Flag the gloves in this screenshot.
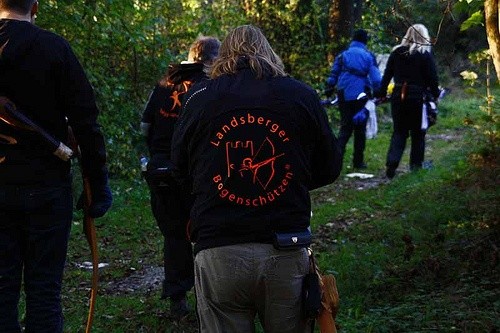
[379,89,388,98]
[324,86,335,98]
[373,92,380,99]
[75,171,113,219]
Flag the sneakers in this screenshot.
[353,163,368,169]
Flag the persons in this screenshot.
[323,28,383,169]
[170,26,343,333]
[379,24,441,178]
[140,36,223,320]
[0,0,112,333]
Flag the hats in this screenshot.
[353,29,369,44]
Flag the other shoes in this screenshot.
[409,163,424,171]
[386,161,396,180]
[172,307,193,323]
